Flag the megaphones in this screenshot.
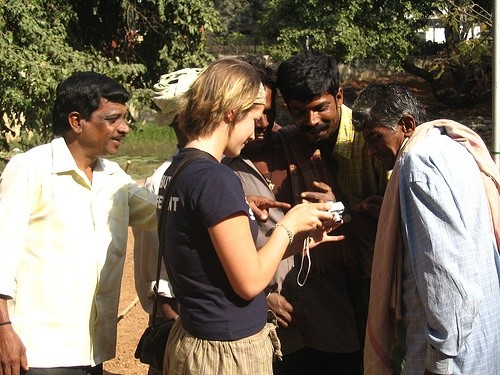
[319,201,345,224]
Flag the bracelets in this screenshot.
[0,71,157,374]
[271,223,295,245]
[1,320,12,327]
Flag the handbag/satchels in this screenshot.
[135,318,176,370]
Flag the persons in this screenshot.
[132,48,291,375]
[277,49,405,375]
[350,77,500,375]
